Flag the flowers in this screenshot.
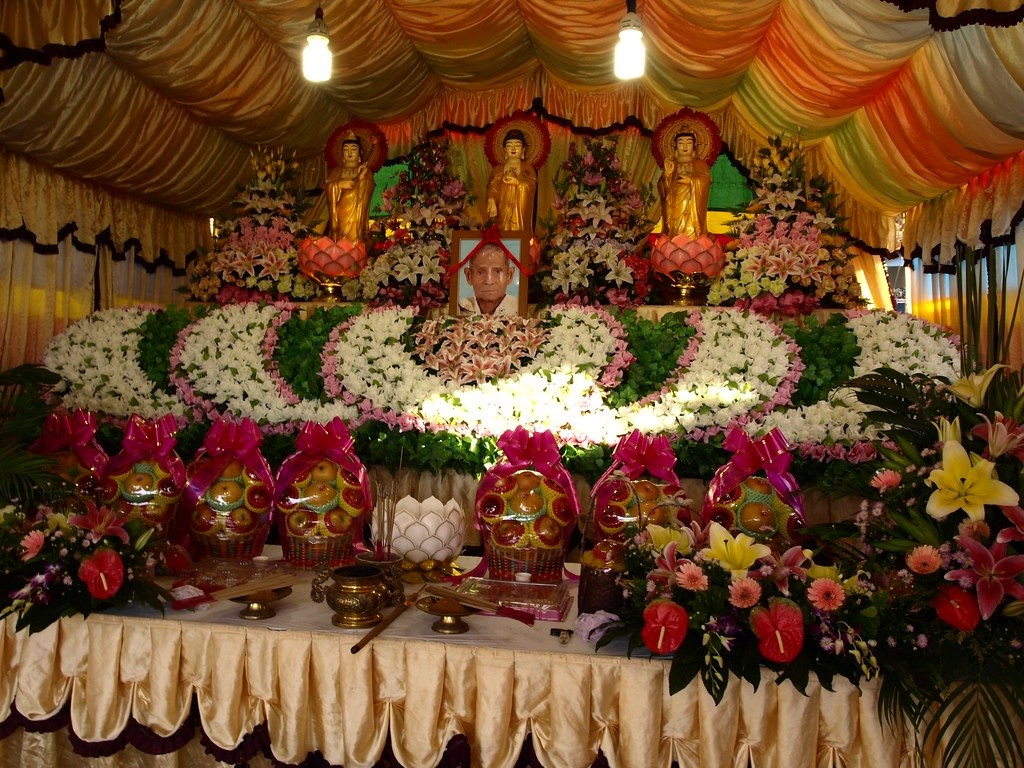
[588,518,880,707]
[0,498,195,638]
[705,127,871,318]
[341,136,468,306]
[827,363,1024,768]
[536,134,654,307]
[172,142,324,302]
[42,304,975,494]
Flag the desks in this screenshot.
[0,556,1024,768]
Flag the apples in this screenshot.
[46,445,368,536]
[477,472,805,565]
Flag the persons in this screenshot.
[486,129,537,231]
[323,130,370,243]
[457,242,518,317]
[657,124,710,241]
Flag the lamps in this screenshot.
[614,0,646,79]
[302,0,333,83]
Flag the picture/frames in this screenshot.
[448,229,530,319]
